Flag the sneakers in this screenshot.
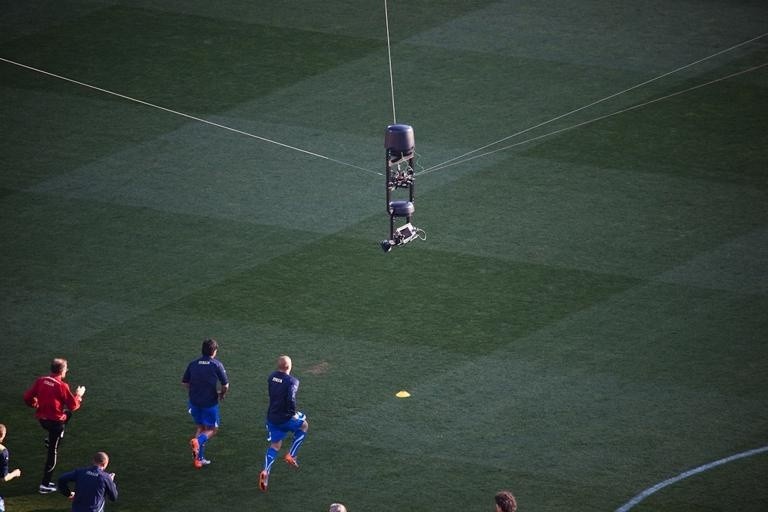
[44,437,49,449]
[191,438,200,460]
[39,481,57,495]
[195,459,211,468]
[285,453,298,468]
[259,470,268,492]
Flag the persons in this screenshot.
[494,491,517,510]
[257,354,309,491]
[22,358,87,495]
[0,421,23,511]
[181,339,230,470]
[54,452,119,512]
[329,503,347,512]
